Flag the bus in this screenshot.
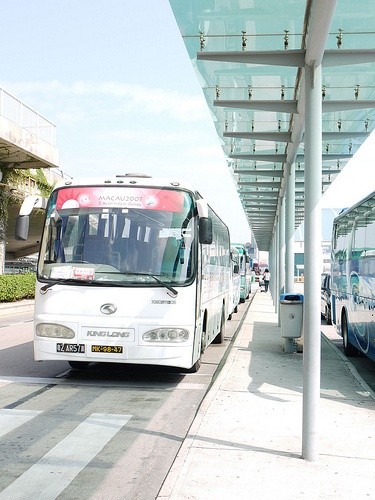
[232,240,254,316]
[34,173,231,375]
[328,191,374,354]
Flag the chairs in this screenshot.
[78,234,191,277]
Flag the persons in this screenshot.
[263,269,270,293]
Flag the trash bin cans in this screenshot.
[279,293,303,338]
